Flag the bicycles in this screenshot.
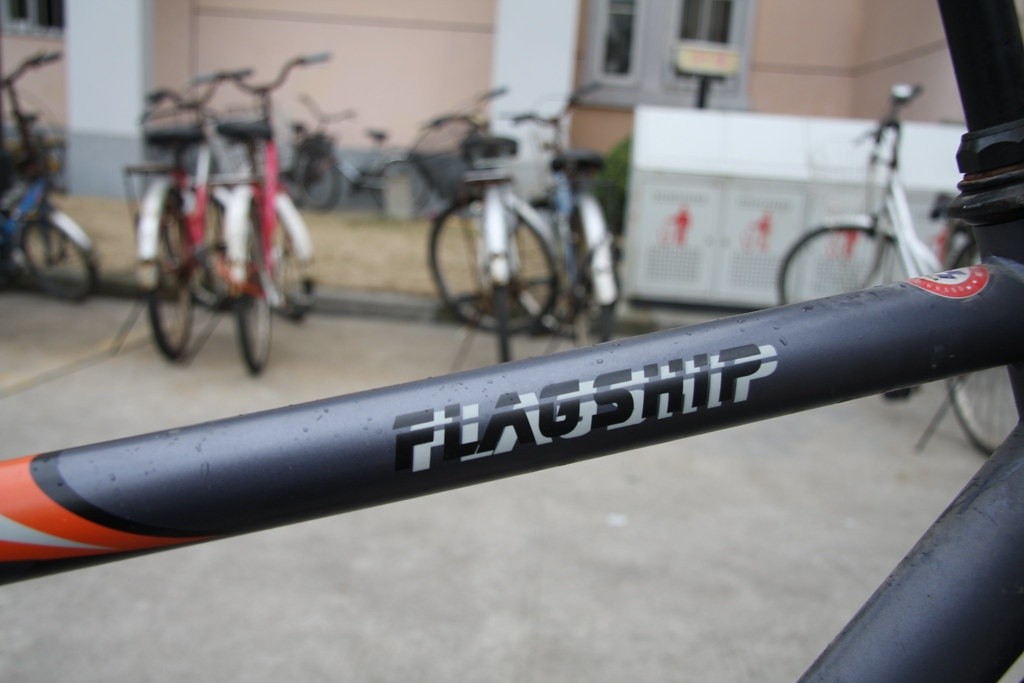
[282,90,433,222]
[375,84,562,363]
[112,41,343,378]
[506,79,619,343]
[775,81,1021,458]
[1,47,100,304]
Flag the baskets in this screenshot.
[142,104,219,175]
[410,119,481,204]
[202,106,294,174]
[306,133,333,155]
[11,138,63,184]
